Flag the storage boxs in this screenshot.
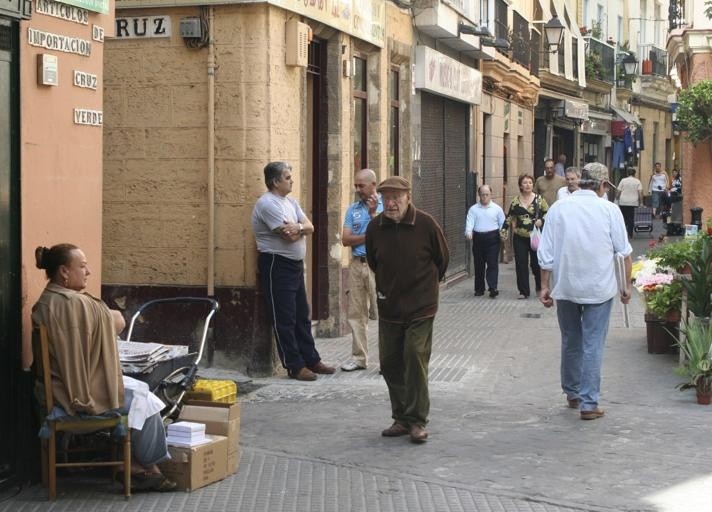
[158,435,228,491]
[176,400,240,476]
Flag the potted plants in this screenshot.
[644,283,681,355]
[658,317,712,405]
[647,236,699,275]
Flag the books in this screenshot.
[167,421,206,431]
[167,431,206,437]
[165,438,206,442]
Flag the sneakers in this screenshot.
[580,407,605,420]
[518,294,528,299]
[409,422,428,442]
[381,421,411,437]
[569,399,580,409]
[340,362,367,371]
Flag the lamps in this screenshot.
[505,14,565,59]
[609,52,640,83]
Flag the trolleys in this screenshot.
[632,206,655,240]
[121,293,221,430]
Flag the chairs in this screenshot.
[29,322,133,500]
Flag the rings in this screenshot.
[286,230,290,234]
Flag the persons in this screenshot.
[534,160,567,205]
[341,169,385,372]
[537,162,633,420]
[557,167,581,201]
[614,168,643,243]
[555,154,566,175]
[648,163,670,218]
[366,176,449,442]
[251,160,336,380]
[465,185,507,297]
[669,167,680,192]
[32,243,177,491]
[501,175,549,300]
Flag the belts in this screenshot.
[352,256,368,263]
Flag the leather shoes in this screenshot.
[288,368,318,381]
[114,471,167,491]
[310,361,336,374]
[474,291,484,296]
[155,478,179,490]
[489,289,499,298]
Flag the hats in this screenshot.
[581,161,617,189]
[375,176,412,192]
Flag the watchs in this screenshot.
[300,223,303,236]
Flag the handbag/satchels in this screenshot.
[500,247,513,264]
